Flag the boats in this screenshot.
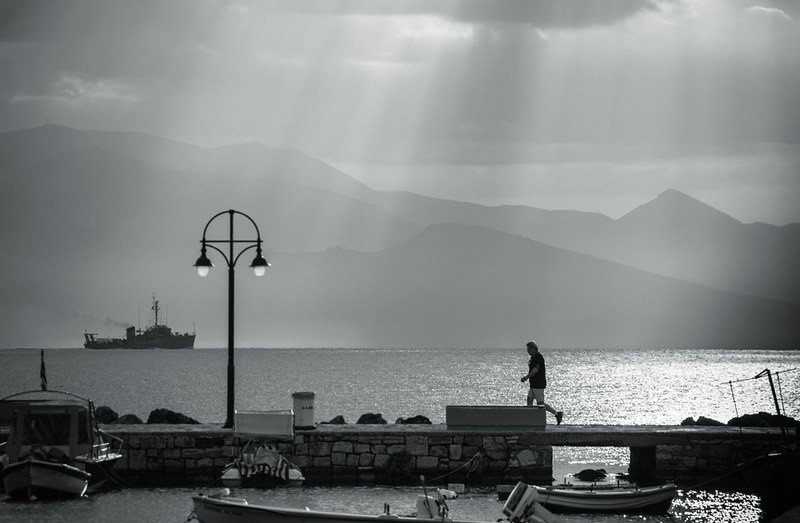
[495,465,679,517]
[183,474,491,523]
[0,346,124,501]
[83,291,196,350]
[1,447,92,502]
[218,435,306,489]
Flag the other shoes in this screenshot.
[554,412,563,425]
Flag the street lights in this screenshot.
[192,209,273,431]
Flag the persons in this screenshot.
[521,341,563,425]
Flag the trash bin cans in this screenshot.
[292,392,315,429]
